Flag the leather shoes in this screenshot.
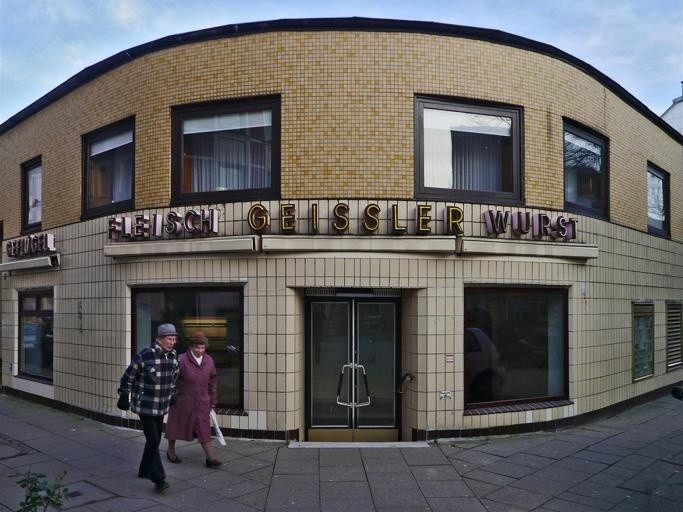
[155,480,169,492]
[205,458,222,468]
[167,451,182,463]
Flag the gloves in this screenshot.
[118,393,130,411]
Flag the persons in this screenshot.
[163,332,222,468]
[117,323,181,493]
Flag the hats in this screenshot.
[190,332,210,345]
[157,323,178,337]
